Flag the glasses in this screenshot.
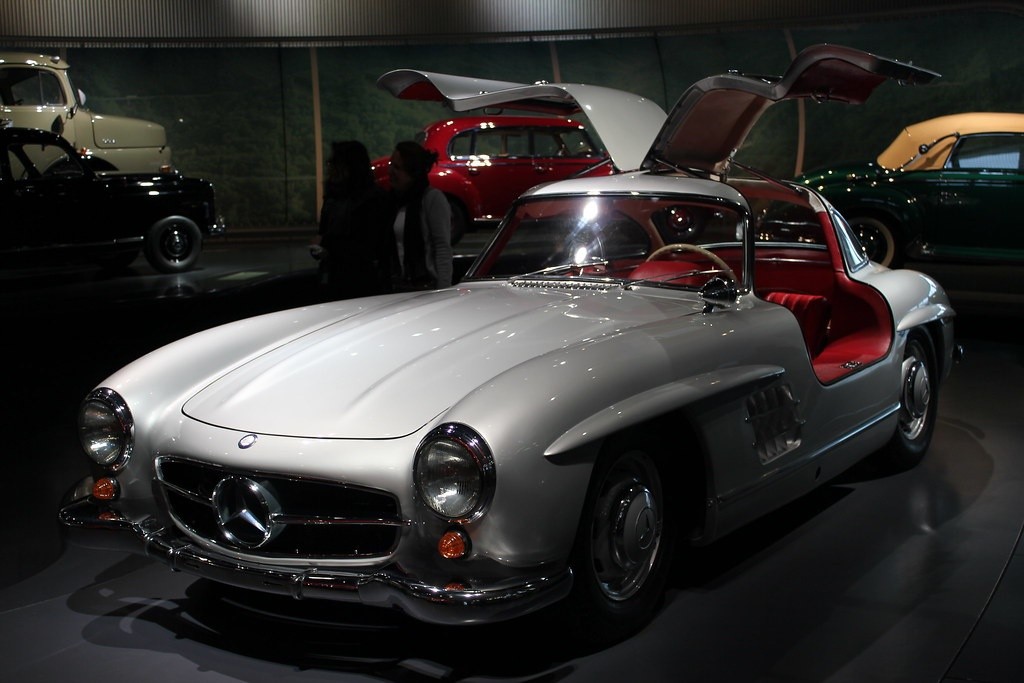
[325,159,345,168]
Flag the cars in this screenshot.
[366,113,617,244]
[1,128,226,279]
[758,110,1024,271]
[0,50,173,177]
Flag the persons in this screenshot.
[309,140,404,303]
[384,141,454,292]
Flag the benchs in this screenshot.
[688,256,858,343]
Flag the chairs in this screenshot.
[629,259,702,288]
[766,292,827,365]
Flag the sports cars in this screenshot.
[55,39,958,629]
